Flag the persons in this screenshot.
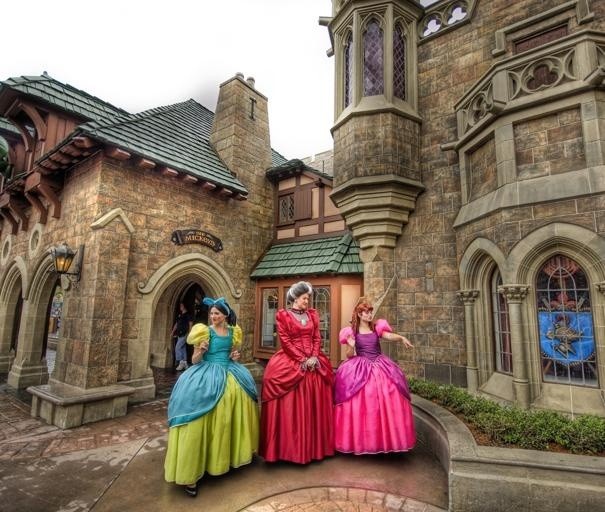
[163,297,260,498]
[192,295,208,326]
[332,297,419,457]
[259,281,337,466]
[168,301,196,372]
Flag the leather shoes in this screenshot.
[184,486,197,496]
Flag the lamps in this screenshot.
[44,241,84,281]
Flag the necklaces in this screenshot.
[291,308,308,327]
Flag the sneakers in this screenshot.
[175,360,189,371]
